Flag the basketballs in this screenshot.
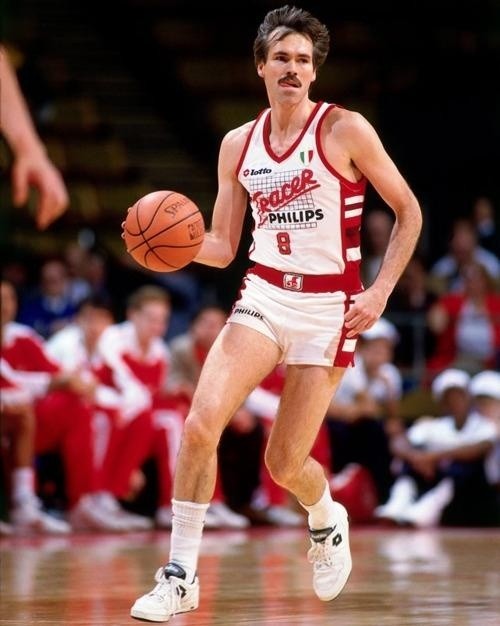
[124,190,205,273]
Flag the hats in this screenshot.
[359,317,499,398]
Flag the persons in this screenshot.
[0,192,498,537]
[127,8,425,622]
[0,45,72,230]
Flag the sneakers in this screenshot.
[0,501,71,537]
[129,563,200,621]
[307,501,353,602]
